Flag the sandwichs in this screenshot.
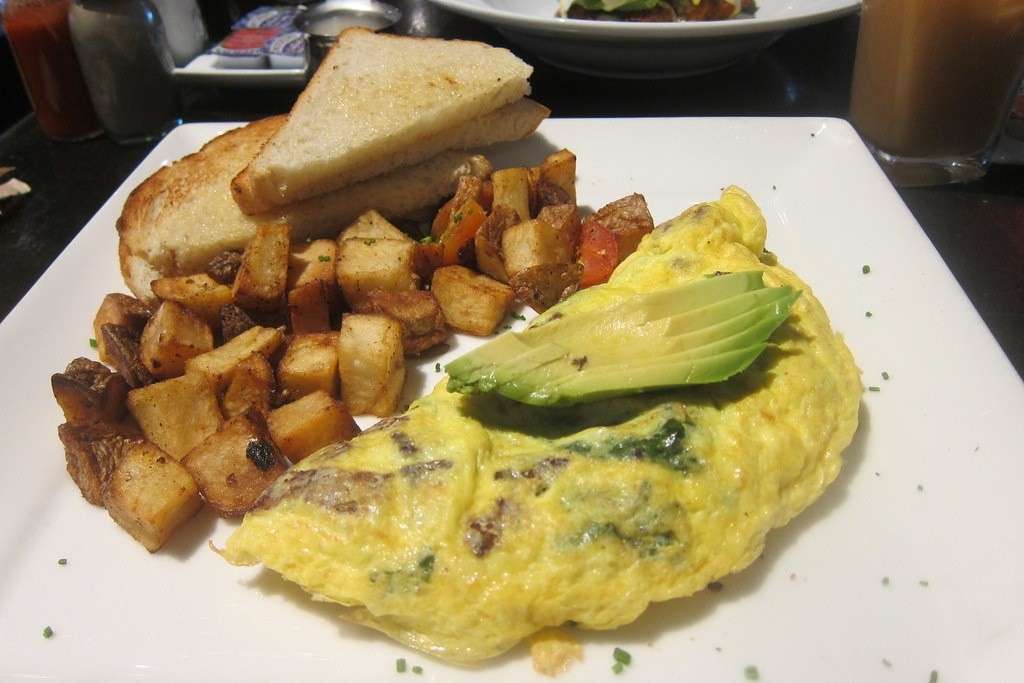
[113,22,551,310]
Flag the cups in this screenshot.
[294,1,403,86]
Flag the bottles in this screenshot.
[0,0,104,142]
[67,0,183,143]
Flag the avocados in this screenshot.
[443,269,802,404]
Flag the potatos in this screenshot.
[57,146,657,553]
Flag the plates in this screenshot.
[0,116,1024,683]
[433,0,863,78]
[171,46,304,90]
